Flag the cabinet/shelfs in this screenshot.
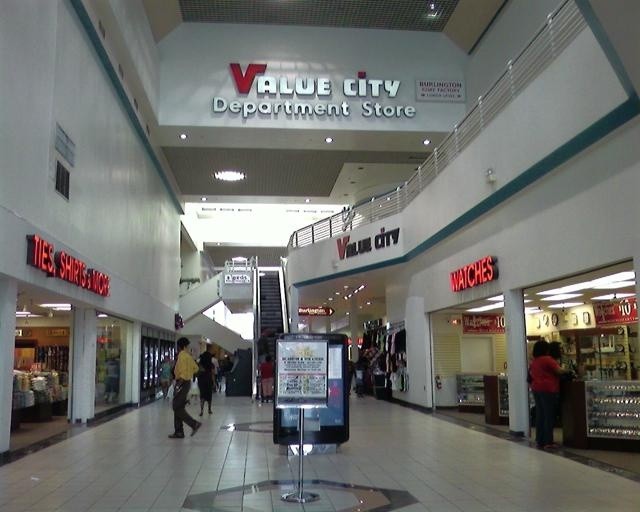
[559,328,638,380]
[456,372,508,425]
[561,380,640,452]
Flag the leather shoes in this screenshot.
[168,432,184,438]
[191,422,201,436]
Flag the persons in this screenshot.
[528,341,576,452]
[158,337,233,438]
[347,361,357,395]
[104,360,119,402]
[261,356,275,403]
